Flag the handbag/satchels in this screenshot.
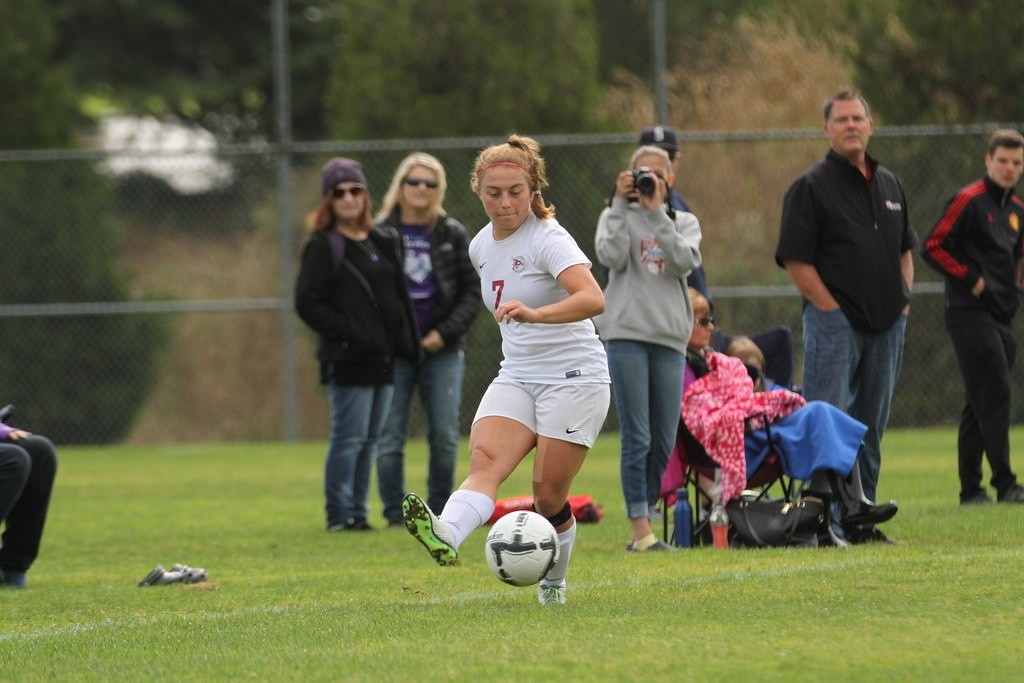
[726,477,832,547]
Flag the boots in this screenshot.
[825,458,897,541]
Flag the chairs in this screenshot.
[711,325,803,503]
[664,365,791,552]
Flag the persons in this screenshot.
[375,151,481,529]
[774,90,920,505]
[403,133,612,609]
[593,146,703,555]
[293,157,423,533]
[0,424,58,591]
[922,130,1024,504]
[683,286,898,546]
[729,335,788,391]
[639,125,713,311]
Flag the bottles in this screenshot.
[709,504,730,551]
[674,488,693,548]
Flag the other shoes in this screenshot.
[961,497,992,503]
[845,529,888,544]
[997,484,1024,501]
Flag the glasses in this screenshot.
[404,177,438,188]
[693,317,715,327]
[330,187,364,198]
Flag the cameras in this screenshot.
[631,166,655,194]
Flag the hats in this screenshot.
[640,126,678,152]
[322,157,367,198]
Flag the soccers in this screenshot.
[485,510,561,587]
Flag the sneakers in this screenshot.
[539,584,565,607]
[400,493,458,566]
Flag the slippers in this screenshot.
[627,540,676,551]
